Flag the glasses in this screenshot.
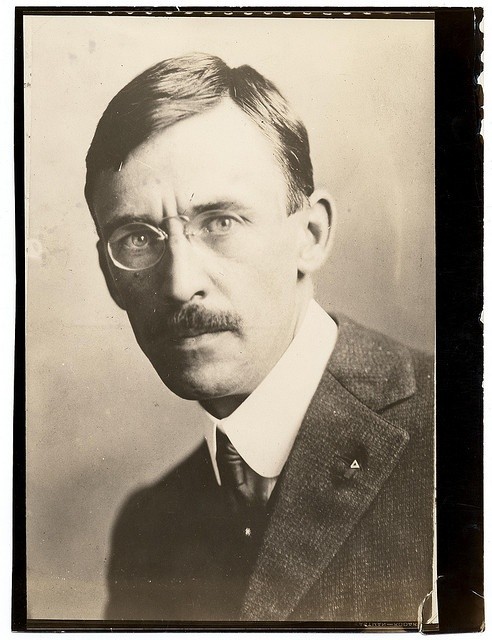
[105,209,251,272]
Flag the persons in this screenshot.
[84,54,434,621]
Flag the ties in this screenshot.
[204,430,268,620]
